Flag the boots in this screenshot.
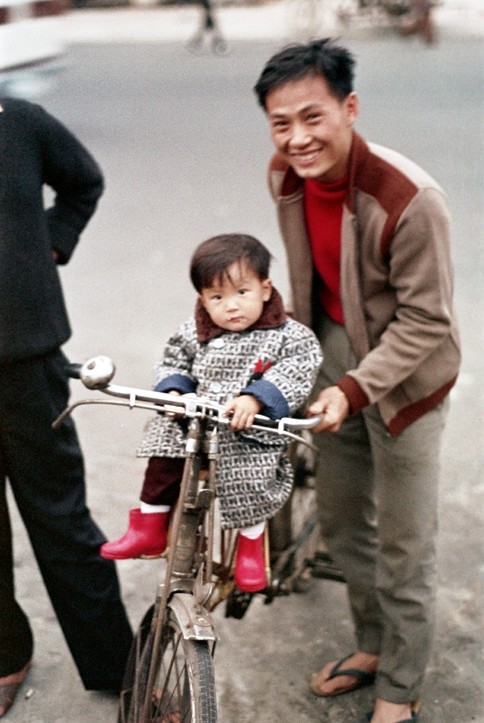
[100,506,171,560]
[234,530,268,590]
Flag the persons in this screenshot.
[0,93,144,699]
[98,233,327,591]
[250,38,462,723]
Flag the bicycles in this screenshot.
[45,354,353,723]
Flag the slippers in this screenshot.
[311,647,376,697]
[363,688,423,722]
